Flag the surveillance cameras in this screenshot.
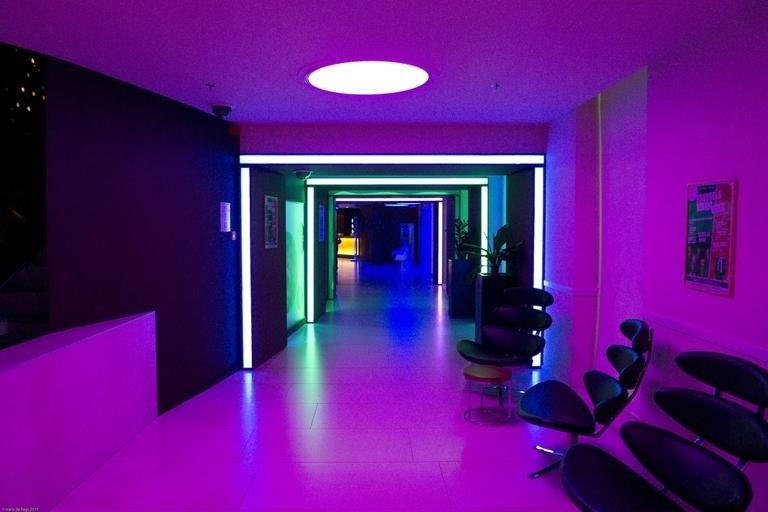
[214,106,231,121]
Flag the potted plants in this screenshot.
[444,217,526,345]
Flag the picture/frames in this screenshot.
[679,175,739,298]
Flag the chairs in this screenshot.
[516,317,653,478]
[559,350,768,511]
[455,285,554,404]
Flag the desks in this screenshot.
[0,311,157,512]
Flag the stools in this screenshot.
[464,365,512,427]
